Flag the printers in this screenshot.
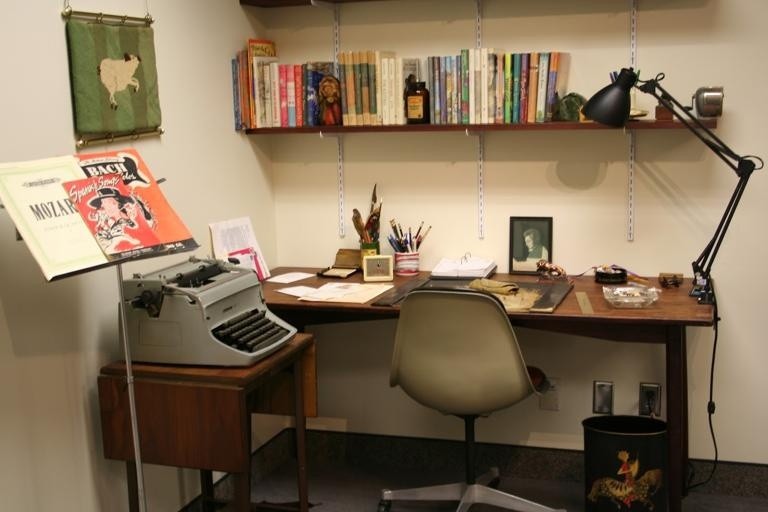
[119,256,297,367]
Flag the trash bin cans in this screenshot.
[582,415,670,512]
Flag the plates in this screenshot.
[595,268,627,283]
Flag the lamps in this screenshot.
[582,67,756,304]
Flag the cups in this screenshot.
[394,252,420,277]
[359,241,380,272]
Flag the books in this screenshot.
[430,252,498,279]
[231,36,561,131]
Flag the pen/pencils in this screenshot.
[387,218,432,253]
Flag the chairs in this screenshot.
[379,288,565,511]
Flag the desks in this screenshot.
[97,332,317,512]
[260,267,719,512]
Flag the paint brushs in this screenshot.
[352,184,383,244]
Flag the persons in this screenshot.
[513,229,548,271]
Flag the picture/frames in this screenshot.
[509,217,553,276]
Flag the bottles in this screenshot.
[404,81,431,124]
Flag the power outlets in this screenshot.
[638,381,662,419]
[539,378,562,411]
